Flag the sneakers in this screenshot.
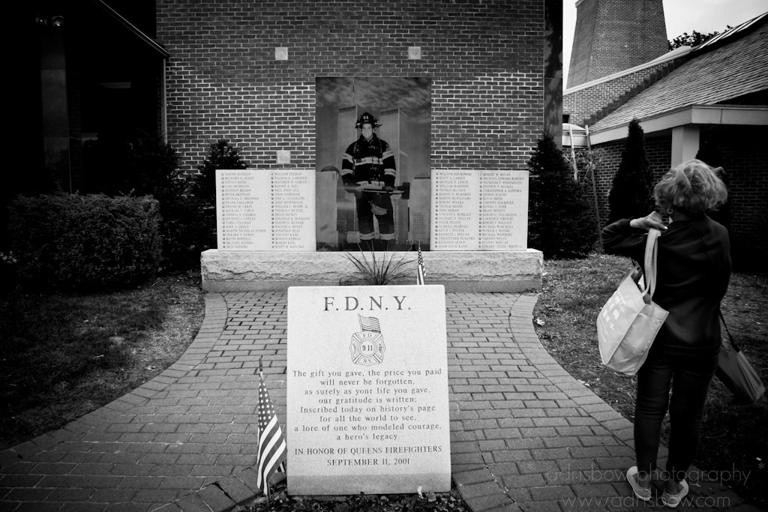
[661,478,690,508]
[625,465,653,502]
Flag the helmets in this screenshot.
[358,112,376,127]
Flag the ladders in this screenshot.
[569,124,603,255]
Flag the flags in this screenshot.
[257,374,286,496]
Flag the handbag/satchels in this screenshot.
[595,227,672,378]
[714,309,766,405]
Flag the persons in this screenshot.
[342,112,396,251]
[601,158,731,508]
